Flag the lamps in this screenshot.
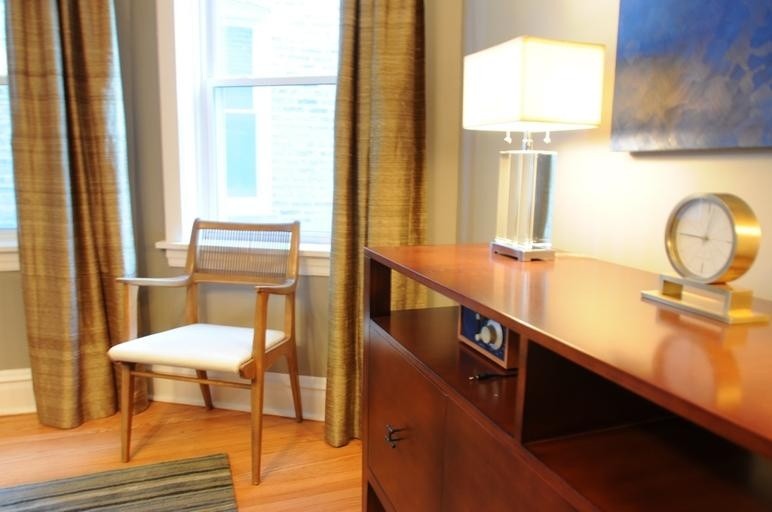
[640,193,771,326]
[460,35,608,263]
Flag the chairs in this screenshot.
[108,219,304,487]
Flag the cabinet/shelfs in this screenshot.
[361,245,772,510]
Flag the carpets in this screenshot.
[1,452,238,512]
